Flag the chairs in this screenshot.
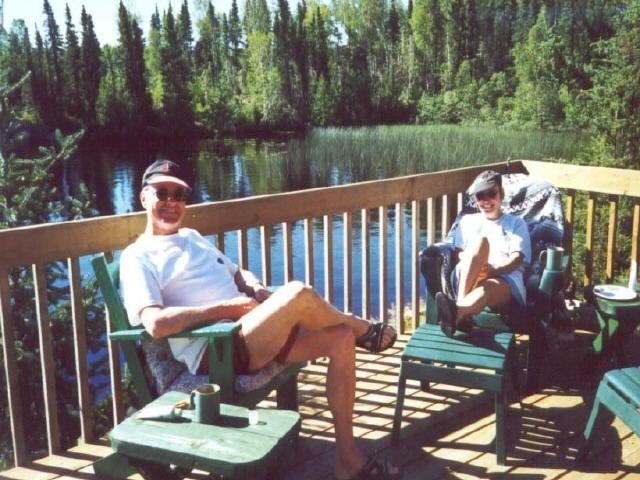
[393,172,566,458]
[90,254,308,456]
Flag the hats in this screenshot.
[142,160,192,190]
[469,169,501,195]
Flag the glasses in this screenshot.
[157,189,186,201]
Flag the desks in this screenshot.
[104,389,301,480]
[573,284,638,367]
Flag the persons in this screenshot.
[118,160,403,479]
[434,169,532,338]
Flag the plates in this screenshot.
[592,285,637,301]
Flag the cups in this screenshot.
[539,246,564,272]
[189,383,221,424]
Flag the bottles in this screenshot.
[248,401,258,425]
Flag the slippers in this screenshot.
[356,323,397,352]
[435,292,456,337]
[355,456,402,480]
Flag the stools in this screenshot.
[577,367,639,472]
